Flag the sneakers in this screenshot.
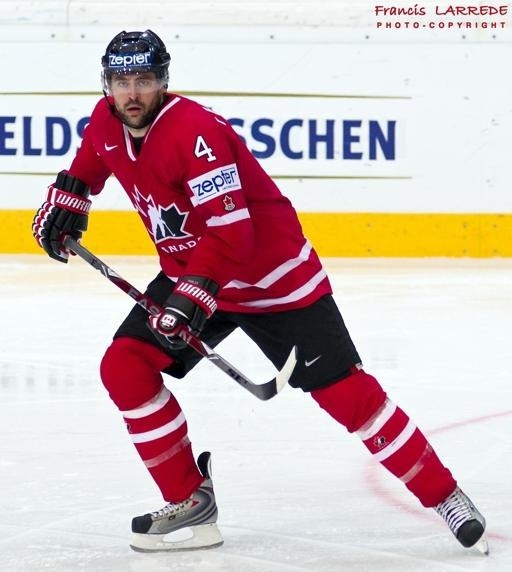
[129,451,220,537]
[432,480,486,549]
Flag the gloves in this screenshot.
[145,270,219,353]
[29,169,93,262]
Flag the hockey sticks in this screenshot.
[63,235,298,400]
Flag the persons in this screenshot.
[29,27,484,546]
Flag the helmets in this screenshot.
[100,29,171,72]
